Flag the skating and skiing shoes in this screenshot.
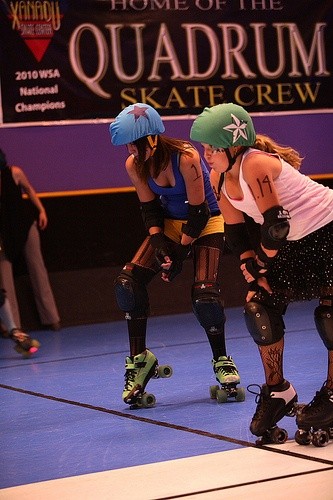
[249,378,299,446]
[10,328,39,358]
[295,386,333,447]
[122,348,173,411]
[209,355,246,403]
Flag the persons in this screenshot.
[0,287,40,358]
[0,148,62,338]
[191,105,332,448]
[109,103,244,411]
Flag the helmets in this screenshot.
[190,102,256,147]
[109,102,165,146]
